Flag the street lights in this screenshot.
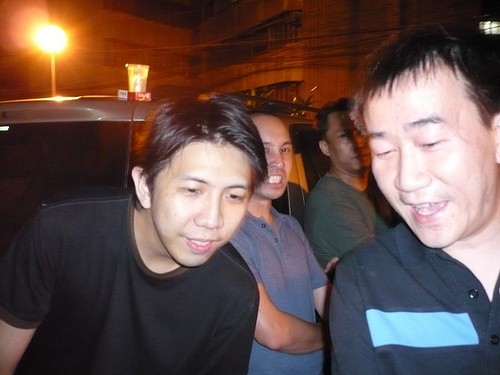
[30,21,68,96]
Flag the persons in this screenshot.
[230,111,334,375]
[327,26,500,375]
[305,98,402,281]
[1,97,268,375]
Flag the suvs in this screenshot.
[0,94,402,264]
